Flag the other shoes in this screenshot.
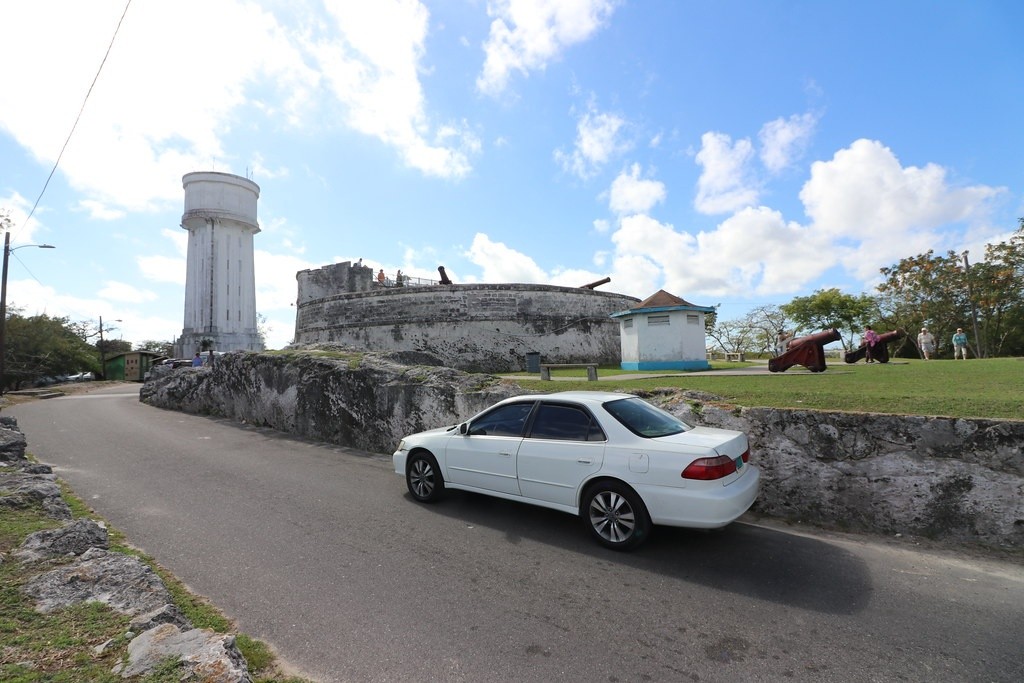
[955,356,958,360]
[864,361,868,365]
[963,356,966,360]
[869,361,875,365]
[924,357,929,361]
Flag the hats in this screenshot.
[776,329,786,334]
[957,328,962,331]
[921,328,928,332]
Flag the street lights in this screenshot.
[99,315,122,381]
[0,230,56,398]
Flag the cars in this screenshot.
[391,389,762,556]
[161,350,225,370]
[36,371,96,387]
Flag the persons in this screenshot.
[952,328,968,360]
[192,350,215,367]
[395,270,405,287]
[774,328,794,355]
[357,258,362,268]
[378,269,385,282]
[863,325,879,365]
[917,327,936,361]
